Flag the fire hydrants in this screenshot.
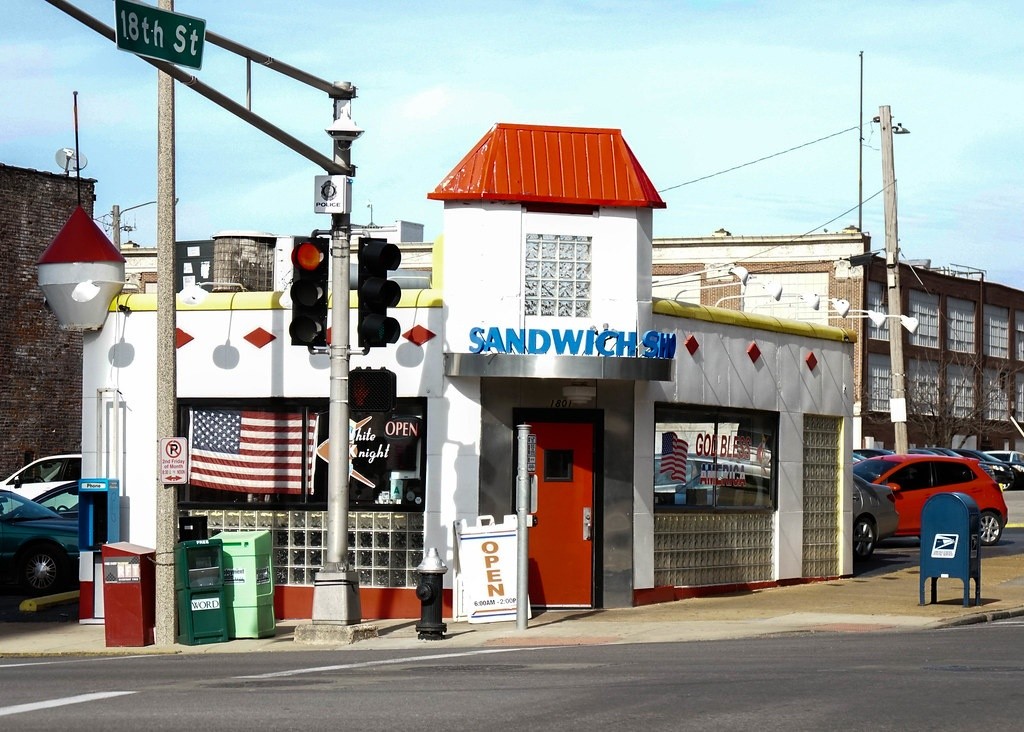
[413,548,450,640]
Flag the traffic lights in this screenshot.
[288,236,329,350]
[357,237,406,353]
[350,366,398,415]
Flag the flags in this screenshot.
[188,408,320,496]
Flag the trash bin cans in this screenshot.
[208,530,276,639]
[918,492,983,606]
[170,539,228,645]
[100,540,157,647]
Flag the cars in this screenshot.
[1,490,79,596]
[852,444,1024,491]
[851,471,901,562]
[1,454,82,512]
[3,482,79,526]
[852,454,1009,545]
